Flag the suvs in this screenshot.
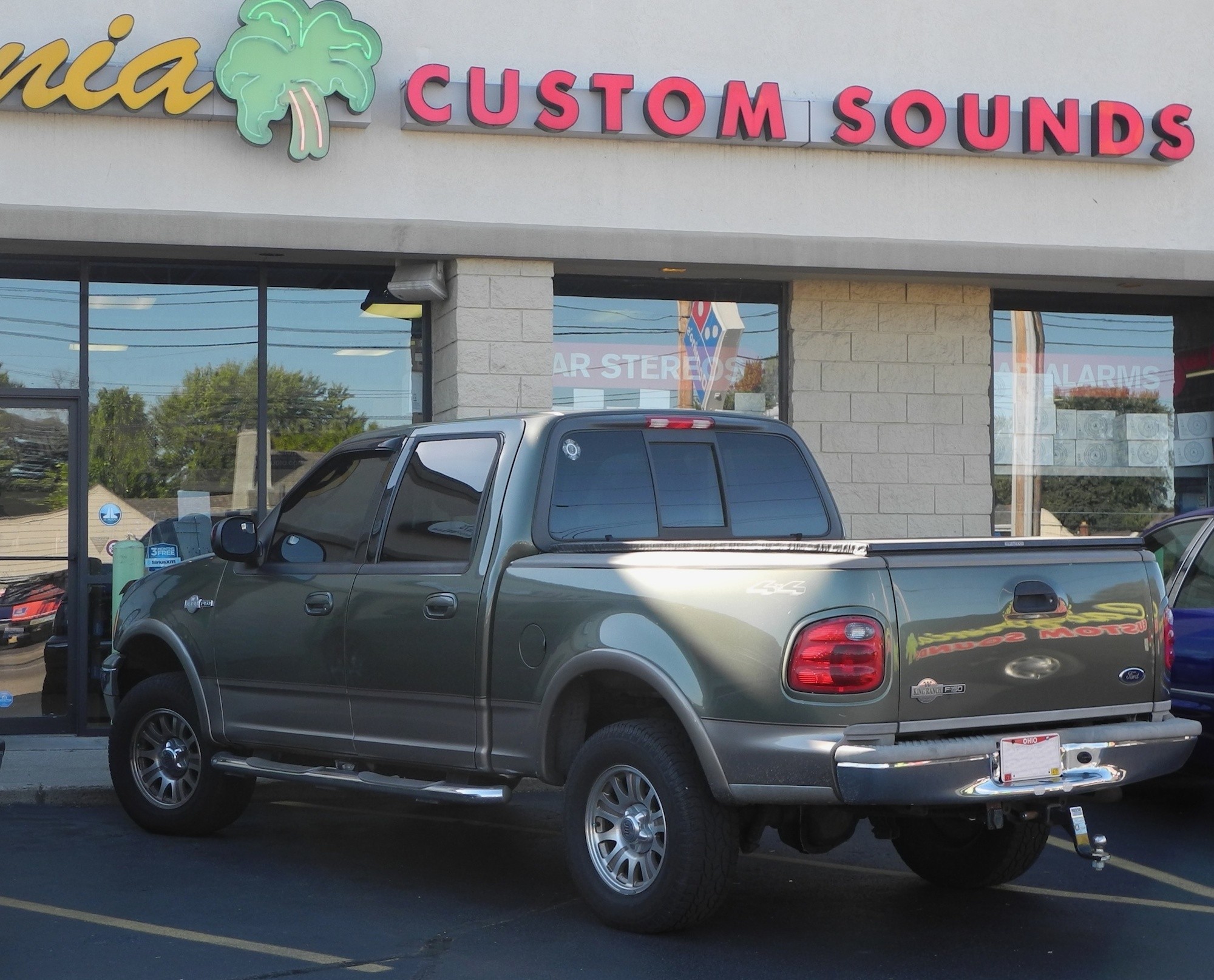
[0,443,250,723]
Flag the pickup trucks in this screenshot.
[111,400,1199,919]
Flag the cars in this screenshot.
[1131,497,1214,818]
